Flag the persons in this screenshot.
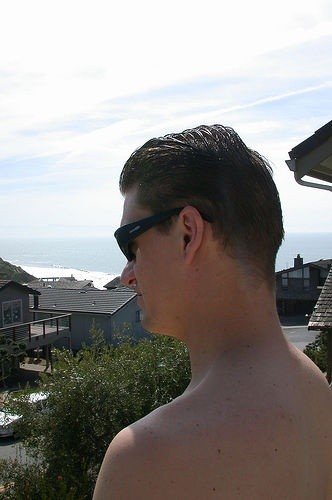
[90,123,332,500]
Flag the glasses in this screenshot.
[114,206,214,262]
